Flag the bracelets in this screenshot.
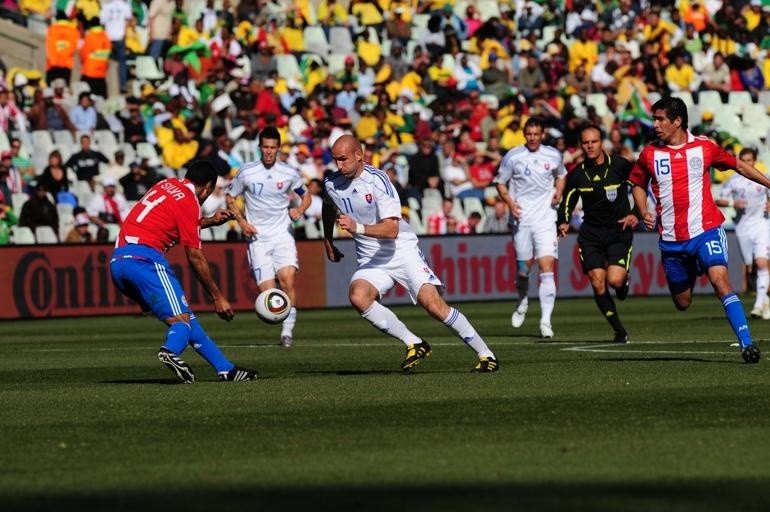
[355,223,365,235]
[729,200,735,207]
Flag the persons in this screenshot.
[557,126,648,344]
[714,149,770,321]
[223,128,312,348]
[630,96,770,364]
[493,116,567,338]
[320,133,499,372]
[0,1,770,246]
[110,159,262,381]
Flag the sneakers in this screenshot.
[402,338,433,372]
[539,323,554,339]
[612,333,628,345]
[615,280,629,300]
[470,354,499,373]
[156,335,292,384]
[742,345,761,363]
[511,303,528,329]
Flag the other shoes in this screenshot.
[746,313,767,321]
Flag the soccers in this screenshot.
[254,288,291,324]
